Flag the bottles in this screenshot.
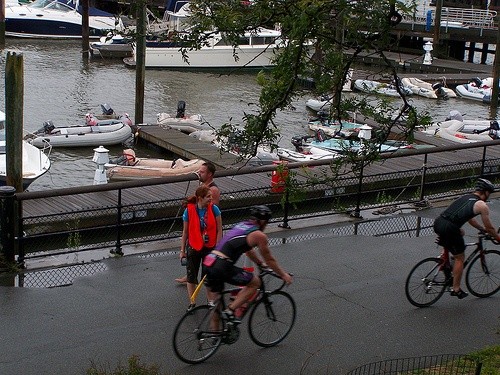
[450,256,454,267]
[234,302,251,323]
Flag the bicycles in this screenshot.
[404,231,500,309]
[171,264,299,366]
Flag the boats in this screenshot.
[354,78,413,96]
[102,154,208,184]
[306,98,366,137]
[0,1,116,39]
[420,110,500,144]
[29,122,131,147]
[0,112,52,190]
[273,137,395,161]
[400,77,458,101]
[91,3,321,70]
[455,77,494,101]
[156,110,280,163]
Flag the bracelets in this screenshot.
[180,250,185,253]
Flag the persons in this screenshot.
[174,163,293,338]
[434,178,500,297]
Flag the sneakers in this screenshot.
[451,288,469,296]
[187,304,196,312]
[222,306,241,324]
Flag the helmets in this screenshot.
[475,179,494,194]
[251,205,272,220]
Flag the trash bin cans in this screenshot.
[271,161,290,192]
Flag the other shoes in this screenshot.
[175,276,187,283]
